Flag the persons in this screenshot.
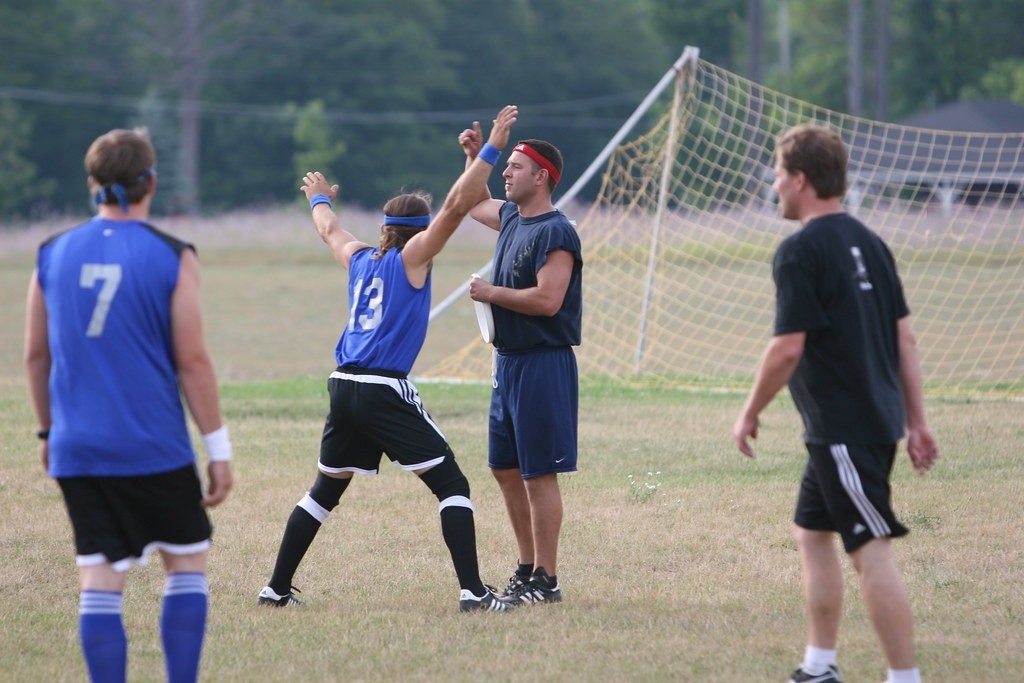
[733,124,942,683]
[26,128,233,683]
[457,120,583,608]
[254,105,522,612]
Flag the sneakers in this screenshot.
[498,580,561,606]
[790,664,840,683]
[258,586,303,608]
[500,574,531,597]
[460,586,513,611]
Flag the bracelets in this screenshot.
[309,193,332,210]
[477,142,503,167]
[36,430,50,440]
